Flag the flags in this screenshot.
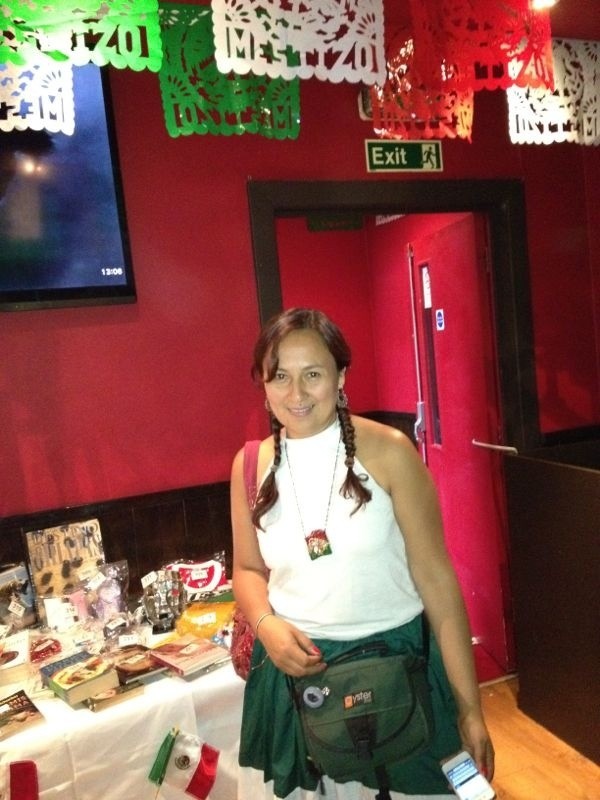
[149,728,220,800]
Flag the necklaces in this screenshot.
[284,429,341,560]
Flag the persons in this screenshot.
[231,308,494,800]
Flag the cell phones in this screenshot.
[440,750,497,800]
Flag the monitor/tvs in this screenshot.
[0,45,137,311]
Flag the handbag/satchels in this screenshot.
[289,641,437,784]
[228,439,260,681]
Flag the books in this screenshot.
[0,690,45,742]
[0,630,230,713]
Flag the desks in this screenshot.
[0,580,282,800]
